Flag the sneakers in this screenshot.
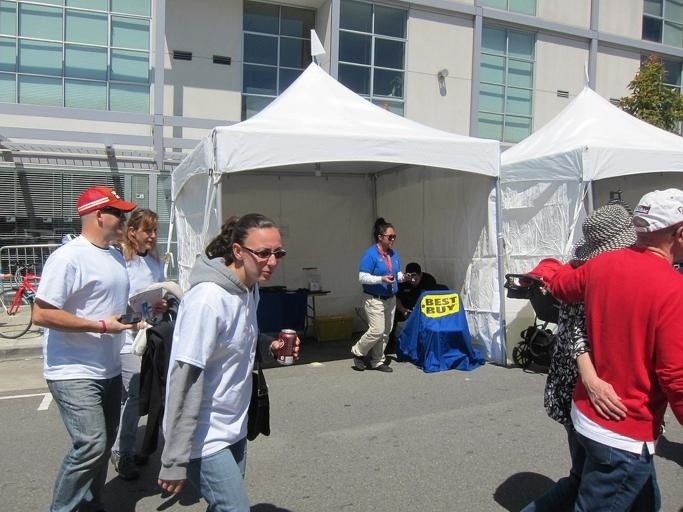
[376,363,393,372]
[111,450,140,479]
[354,352,365,370]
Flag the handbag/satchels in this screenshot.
[247,372,271,441]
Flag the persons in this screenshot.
[110,208,180,482]
[520,188,683,512]
[521,202,628,512]
[157,213,287,512]
[394,262,436,320]
[31,186,143,512]
[350,216,412,373]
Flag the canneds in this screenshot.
[276,329,296,365]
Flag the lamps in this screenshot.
[315,163,321,176]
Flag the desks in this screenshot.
[288,289,332,330]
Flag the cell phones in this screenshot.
[118,312,143,324]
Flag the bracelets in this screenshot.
[98,320,106,333]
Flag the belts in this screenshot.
[363,290,393,299]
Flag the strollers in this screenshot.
[504,257,561,366]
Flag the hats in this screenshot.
[77,186,137,216]
[572,204,638,261]
[634,188,683,233]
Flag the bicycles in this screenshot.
[0,267,42,339]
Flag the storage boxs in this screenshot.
[315,315,353,342]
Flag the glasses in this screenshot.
[383,234,396,241]
[242,247,287,258]
